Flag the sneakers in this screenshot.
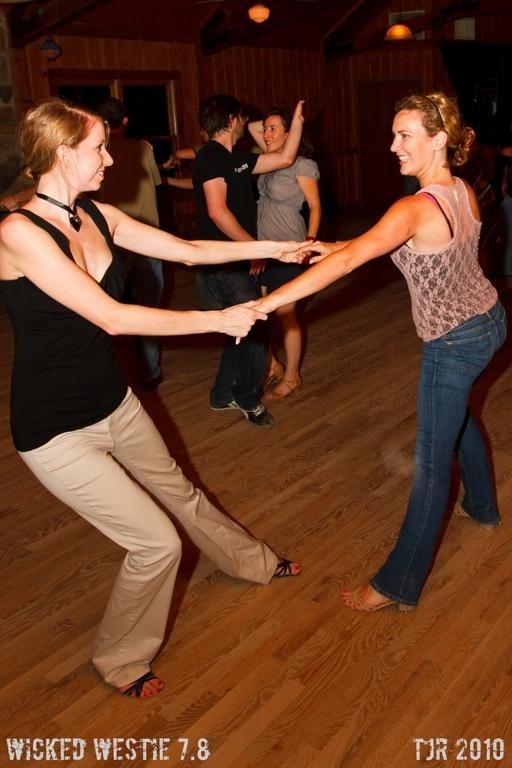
[232,396,274,428]
[209,387,240,410]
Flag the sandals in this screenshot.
[264,375,302,403]
[261,362,284,392]
[123,670,156,698]
[273,558,297,576]
[337,589,415,614]
[453,499,496,530]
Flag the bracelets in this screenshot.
[304,236,316,242]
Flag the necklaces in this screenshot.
[34,191,82,232]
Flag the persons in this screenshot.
[79,99,164,393]
[247,106,322,402]
[158,155,181,170]
[190,93,306,430]
[162,126,210,190]
[1,99,314,700]
[234,91,507,613]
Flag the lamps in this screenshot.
[38,32,62,64]
[382,1,417,42]
